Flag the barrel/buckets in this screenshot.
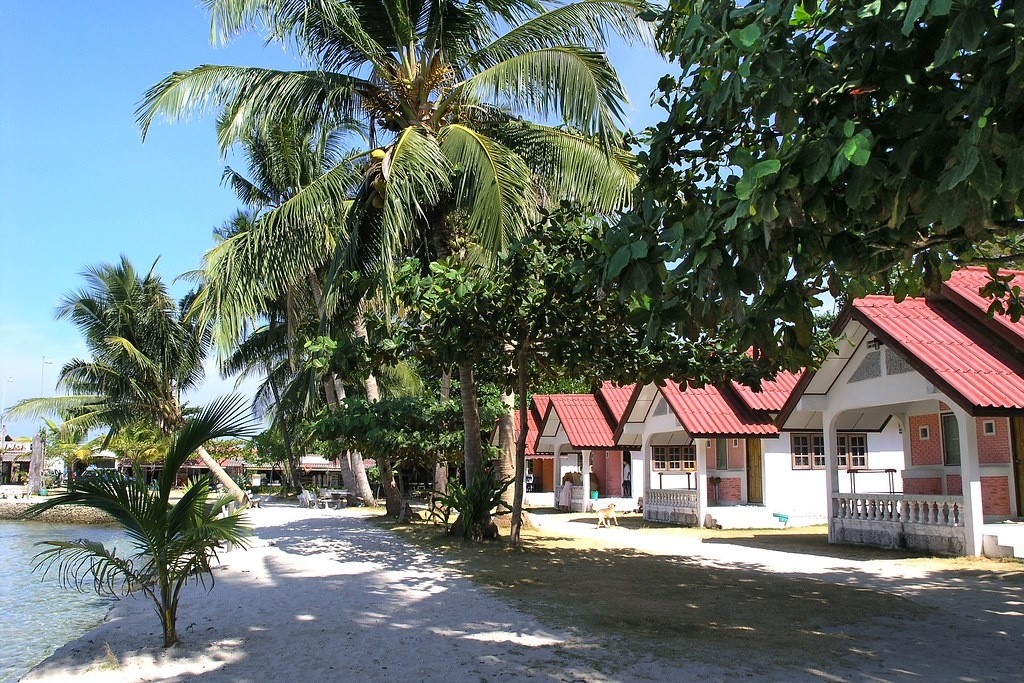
[591,490,598,499]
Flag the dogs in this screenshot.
[597,503,618,529]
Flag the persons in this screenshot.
[559,479,572,513]
[621,458,631,498]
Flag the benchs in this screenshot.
[300,498,340,510]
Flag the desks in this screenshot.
[319,491,347,509]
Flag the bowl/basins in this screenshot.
[773,513,788,525]
[39,490,48,495]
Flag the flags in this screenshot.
[44,357,53,364]
[7,376,15,383]
